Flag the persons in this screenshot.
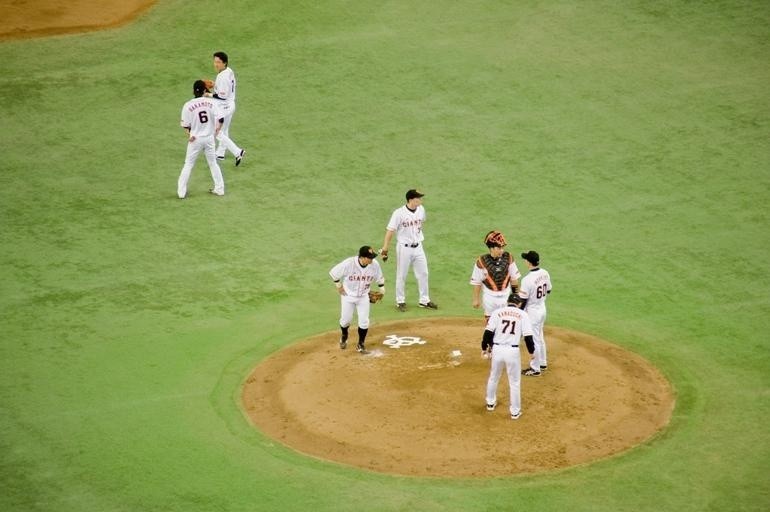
[329,246,385,353]
[205,52,246,166]
[482,294,535,419]
[381,190,437,312]
[517,251,552,376]
[470,230,522,359]
[177,80,224,199]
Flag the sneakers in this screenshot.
[419,302,438,309]
[540,366,547,370]
[521,368,541,376]
[356,343,365,352]
[511,412,521,418]
[487,401,497,410]
[398,303,406,311]
[340,337,347,348]
[217,156,225,160]
[236,150,245,166]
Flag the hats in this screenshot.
[485,231,506,246]
[507,294,521,303]
[521,251,539,262]
[406,190,425,199]
[194,80,205,97]
[360,246,378,258]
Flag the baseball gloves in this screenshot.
[368,291,384,303]
[203,79,214,90]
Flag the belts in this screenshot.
[405,244,419,248]
[493,344,518,347]
